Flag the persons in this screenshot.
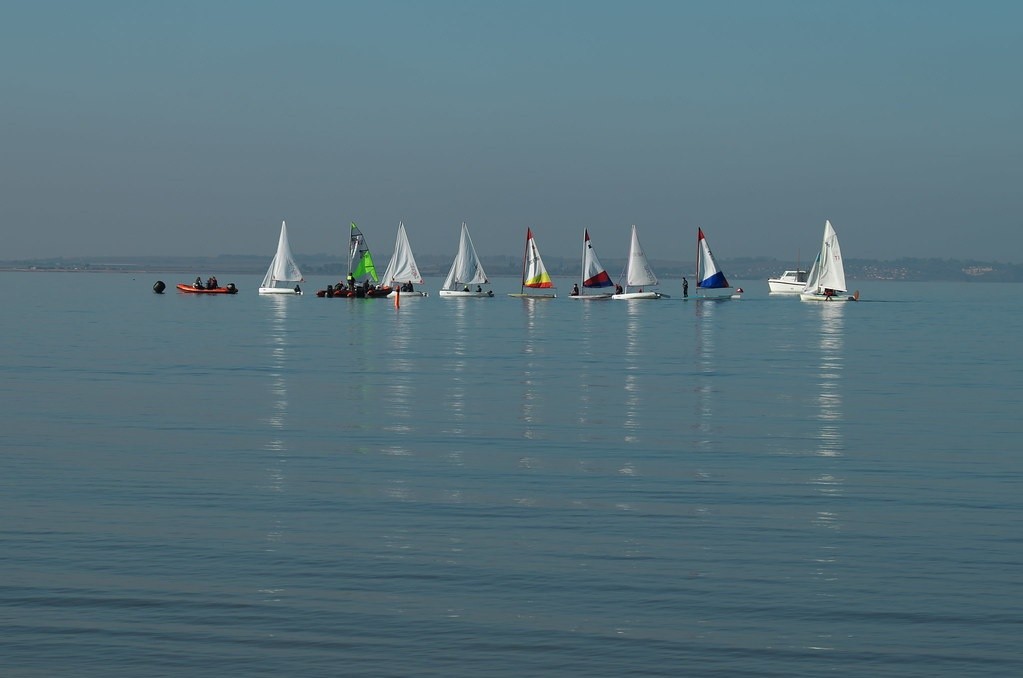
[682,277,688,297]
[401,281,413,292]
[196,277,204,289]
[616,284,622,294]
[463,286,469,291]
[823,289,837,300]
[207,276,218,289]
[295,285,301,292]
[349,272,355,288]
[363,279,369,292]
[476,285,482,292]
[571,284,579,296]
[335,281,343,290]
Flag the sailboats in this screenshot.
[611,224,662,300]
[316,219,393,299]
[507,225,557,299]
[568,227,616,299]
[378,219,429,299]
[439,220,495,298]
[258,218,307,295]
[800,220,859,302]
[680,224,741,301]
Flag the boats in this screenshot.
[768,259,809,295]
[177,283,238,294]
